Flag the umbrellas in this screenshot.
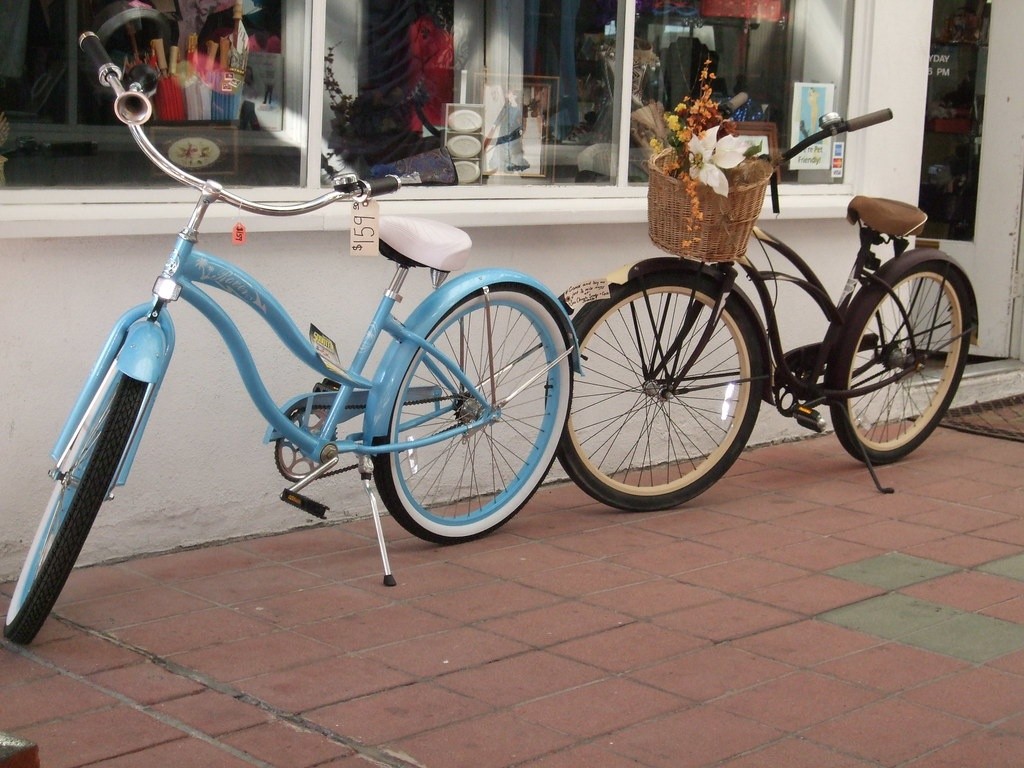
[121,0,246,121]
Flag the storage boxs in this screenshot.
[440,72,559,185]
[148,123,238,182]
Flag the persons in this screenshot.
[239,66,259,130]
[405,0,454,132]
[263,64,275,104]
[495,91,531,172]
[579,35,666,177]
[659,36,719,115]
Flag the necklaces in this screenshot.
[675,42,703,95]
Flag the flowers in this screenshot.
[651,54,765,251]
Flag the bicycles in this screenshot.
[0,30,586,646]
[544,91,981,513]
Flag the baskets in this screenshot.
[647,147,773,261]
[156,76,242,122]
